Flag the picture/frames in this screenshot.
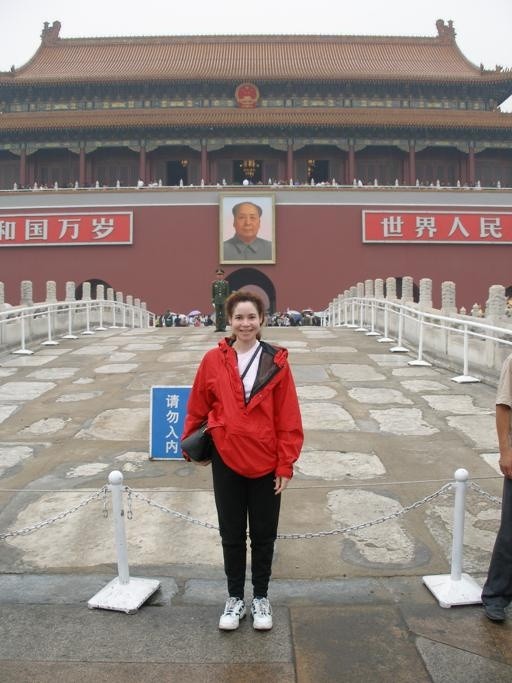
[219,191,276,264]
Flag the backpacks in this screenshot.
[166,315,172,325]
[155,320,160,326]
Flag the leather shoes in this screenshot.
[214,329,227,332]
[484,603,506,621]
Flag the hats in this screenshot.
[215,269,224,274]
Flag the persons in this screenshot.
[216,178,222,185]
[137,176,146,186]
[115,175,121,186]
[273,179,340,185]
[148,307,321,327]
[241,177,250,185]
[211,268,230,333]
[223,201,272,259]
[478,351,510,621]
[255,178,264,184]
[147,174,159,186]
[18,181,48,189]
[357,176,364,184]
[179,288,304,631]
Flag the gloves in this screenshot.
[212,303,216,308]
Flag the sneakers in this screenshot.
[219,597,247,630]
[249,596,273,631]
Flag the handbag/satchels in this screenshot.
[179,422,216,461]
